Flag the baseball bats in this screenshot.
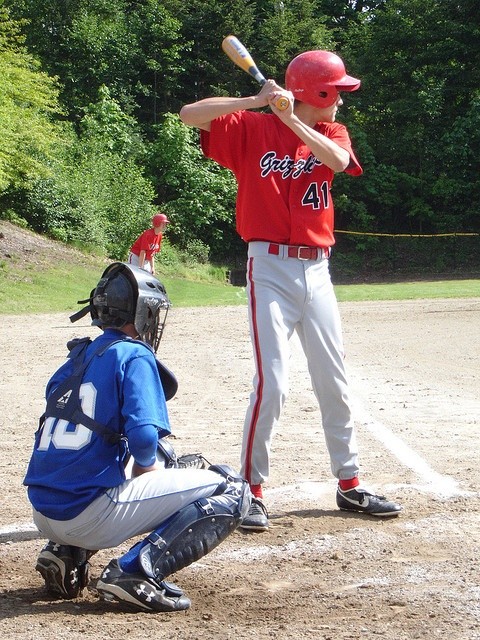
[222,34,289,110]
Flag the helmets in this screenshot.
[153,214,169,227]
[69,262,169,354]
[285,50,360,109]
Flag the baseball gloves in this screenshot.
[172,454,213,469]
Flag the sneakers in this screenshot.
[336,485,402,517]
[241,494,269,532]
[95,558,191,613]
[35,541,81,599]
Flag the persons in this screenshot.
[24,262,251,614]
[130,214,169,276]
[179,49,403,529]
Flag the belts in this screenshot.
[268,244,329,261]
